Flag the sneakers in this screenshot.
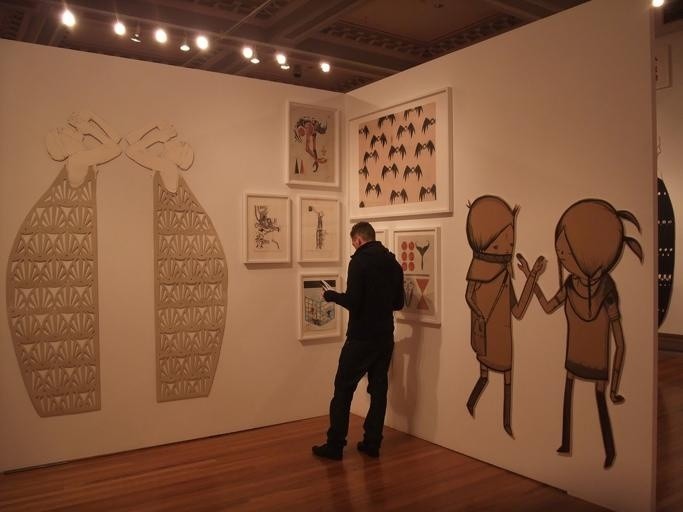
[312,446,343,459]
[358,442,380,457]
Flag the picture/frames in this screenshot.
[285,101,341,192]
[349,86,454,223]
[296,193,342,264]
[243,191,292,265]
[393,226,441,326]
[376,227,390,249]
[298,272,342,341]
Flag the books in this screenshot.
[320,279,347,294]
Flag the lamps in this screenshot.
[130,22,141,43]
[250,44,260,64]
[179,31,190,52]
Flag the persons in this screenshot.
[312,221,404,461]
